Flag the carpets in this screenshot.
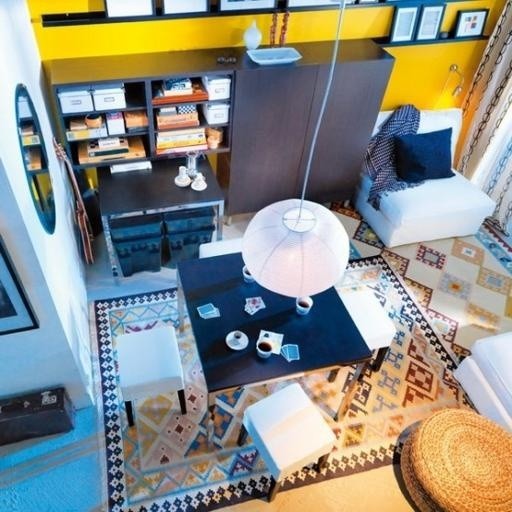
[93,258,486,511]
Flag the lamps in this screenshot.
[240,1,351,301]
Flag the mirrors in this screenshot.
[14,83,56,237]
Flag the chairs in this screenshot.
[455,331,511,434]
[355,105,497,248]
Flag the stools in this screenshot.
[238,383,337,504]
[401,408,512,512]
[327,288,397,382]
[114,325,189,428]
[198,237,247,258]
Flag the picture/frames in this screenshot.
[391,5,420,43]
[0,236,40,334]
[454,10,489,37]
[416,4,446,40]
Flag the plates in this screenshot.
[225,332,249,350]
[249,47,304,65]
[191,182,208,192]
[172,176,191,187]
[258,329,284,356]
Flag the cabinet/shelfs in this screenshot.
[225,34,397,214]
[94,155,226,284]
[42,47,233,172]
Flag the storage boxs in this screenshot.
[57,84,94,114]
[201,72,231,100]
[91,80,130,112]
[202,102,230,125]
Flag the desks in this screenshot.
[176,254,374,422]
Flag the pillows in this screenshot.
[393,128,455,182]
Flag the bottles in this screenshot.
[243,21,262,49]
[186,151,197,177]
[85,114,102,129]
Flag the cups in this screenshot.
[234,331,242,344]
[295,296,314,316]
[193,172,204,187]
[242,265,254,283]
[256,340,273,359]
[178,166,187,181]
